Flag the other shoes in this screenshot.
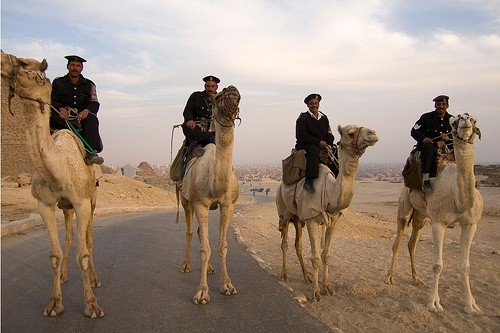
[303,179,316,194]
[84,154,104,166]
[422,180,433,194]
[189,145,205,159]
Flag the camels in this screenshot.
[276,124,379,302]
[384,112,485,316]
[0,52,105,319]
[176,85,241,305]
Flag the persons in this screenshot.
[410,95,457,193]
[295,94,339,193]
[181,76,220,158]
[50,55,104,167]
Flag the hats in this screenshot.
[432,95,449,102]
[64,55,87,62]
[202,76,220,83]
[304,94,322,104]
